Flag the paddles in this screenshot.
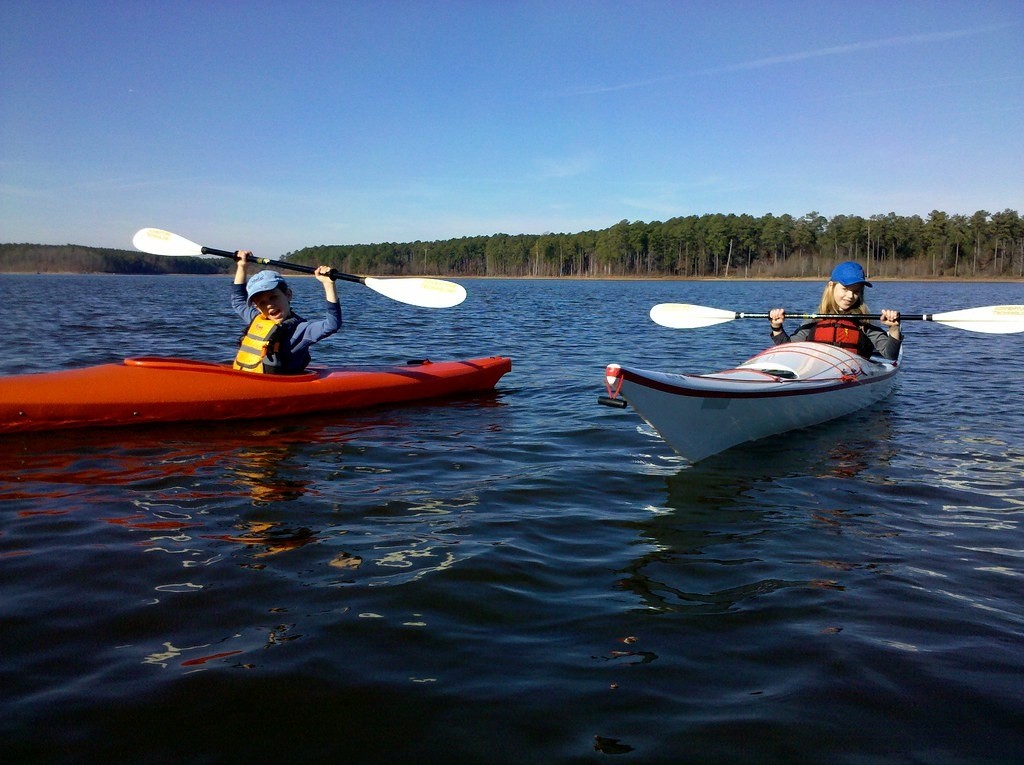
[132,225,468,310]
[649,301,1024,335]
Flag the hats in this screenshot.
[246,270,287,307]
[831,262,873,287]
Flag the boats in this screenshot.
[596,342,903,464]
[0,355,512,437]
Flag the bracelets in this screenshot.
[771,324,782,332]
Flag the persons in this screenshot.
[769,261,904,365]
[231,250,342,375]
